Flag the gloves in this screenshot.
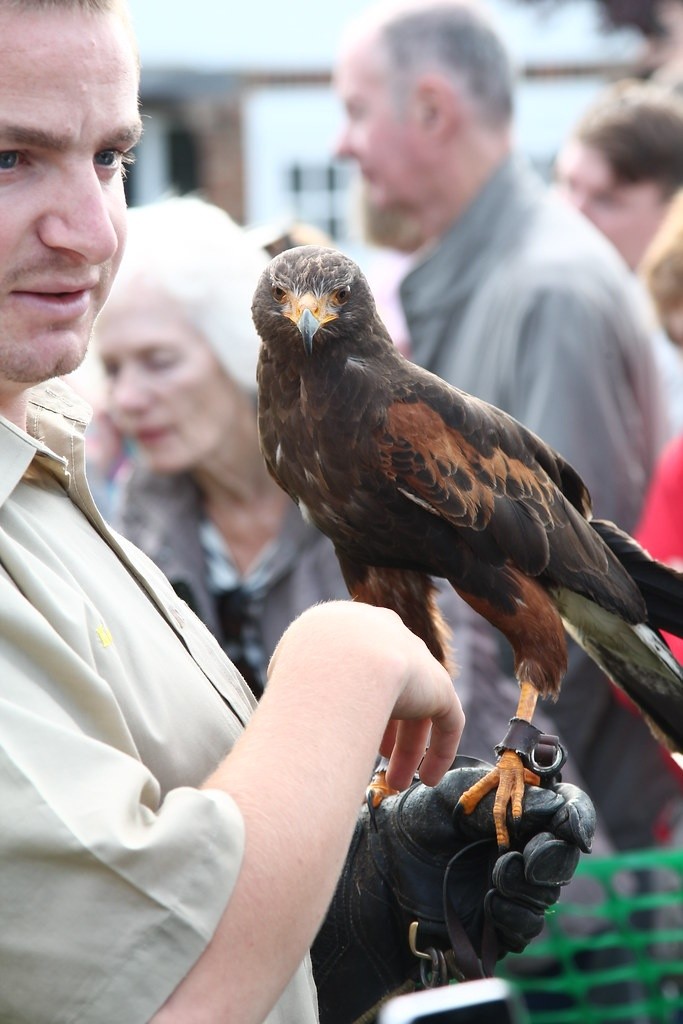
[306,757,597,1024]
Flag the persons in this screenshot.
[86,191,642,948]
[0,3,468,1024]
[314,0,682,773]
